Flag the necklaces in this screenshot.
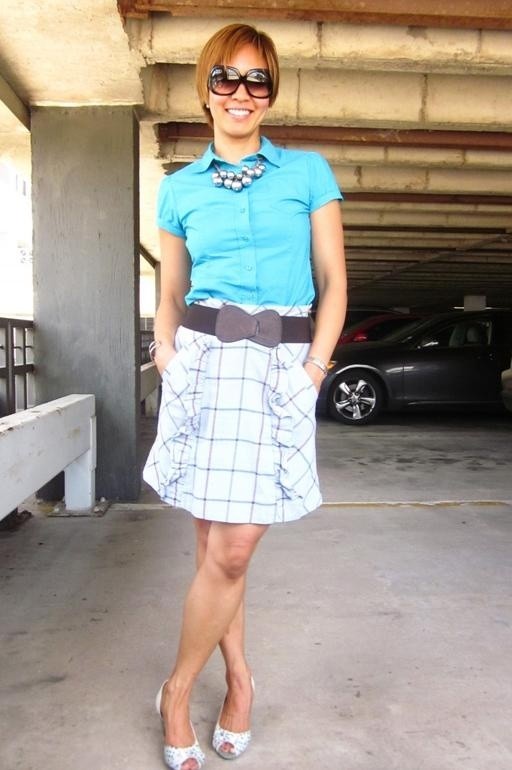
[211,156,267,192]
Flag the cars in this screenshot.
[315,307,511,426]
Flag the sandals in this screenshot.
[153,678,204,770]
[212,677,257,761]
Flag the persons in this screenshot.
[140,22,353,770]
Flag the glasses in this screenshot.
[207,63,273,99]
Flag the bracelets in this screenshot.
[303,355,329,378]
[148,335,172,361]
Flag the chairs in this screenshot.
[463,324,488,346]
